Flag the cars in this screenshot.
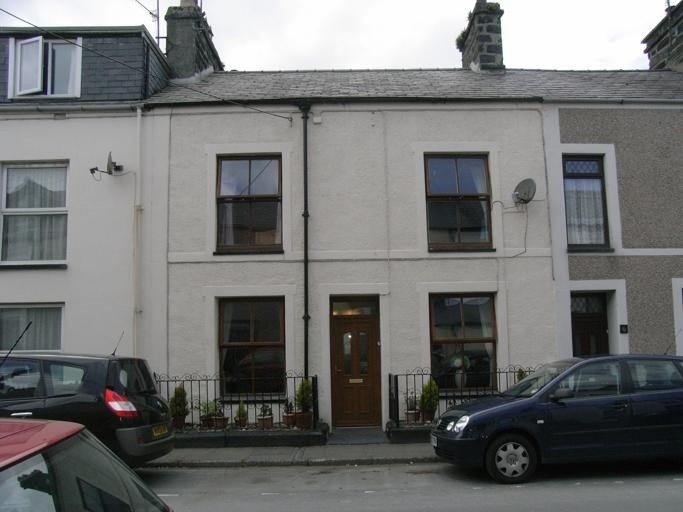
[431,351,490,387]
[428,355,683,485]
[0,350,176,512]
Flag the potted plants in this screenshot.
[168,375,314,429]
[406,378,440,426]
